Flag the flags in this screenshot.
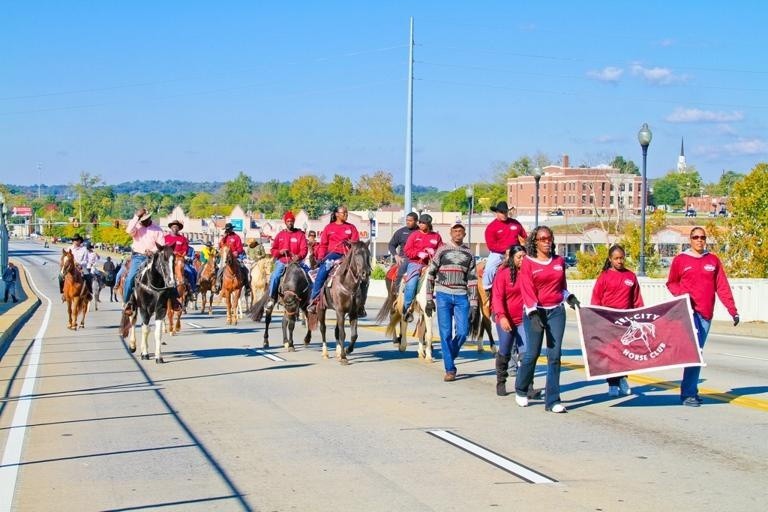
[574,293,706,381]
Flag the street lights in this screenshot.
[301,222,308,236]
[466,185,474,248]
[0,191,11,278]
[36,161,44,199]
[637,123,651,276]
[242,227,247,246]
[368,211,374,263]
[532,166,544,224]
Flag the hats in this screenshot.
[138,208,153,221]
[222,222,236,229]
[168,220,184,232]
[490,198,515,213]
[284,211,296,224]
[71,233,84,243]
[247,237,257,246]
[84,242,93,250]
[449,217,469,230]
[418,214,433,224]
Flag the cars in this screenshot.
[656,257,670,266]
[718,210,728,217]
[56,237,132,256]
[552,209,563,216]
[382,254,393,263]
[671,208,685,213]
[474,255,481,261]
[685,210,696,217]
[562,252,577,266]
[31,231,41,238]
[709,211,716,216]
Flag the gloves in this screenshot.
[731,314,740,326]
[530,312,544,332]
[569,296,582,311]
[425,300,436,317]
[468,300,478,323]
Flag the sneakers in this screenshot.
[549,404,567,414]
[683,397,702,408]
[444,370,457,381]
[516,393,528,406]
[608,378,633,396]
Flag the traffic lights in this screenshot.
[115,220,119,229]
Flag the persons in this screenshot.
[514,226,580,412]
[2,262,19,303]
[665,226,739,407]
[590,245,644,397]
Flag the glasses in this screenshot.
[536,235,554,241]
[691,234,707,240]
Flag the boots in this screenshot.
[494,349,511,396]
[521,360,542,400]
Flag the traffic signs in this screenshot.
[100,222,112,226]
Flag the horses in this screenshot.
[88,240,319,336]
[246,262,312,352]
[376,260,497,364]
[119,241,177,363]
[308,239,371,365]
[60,248,88,330]
